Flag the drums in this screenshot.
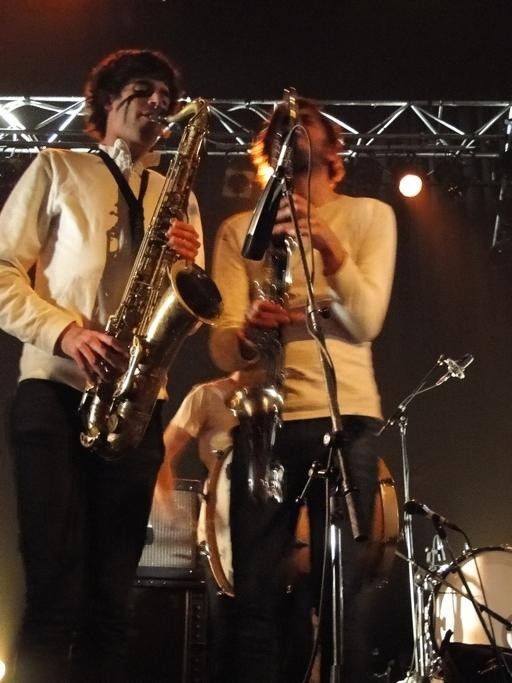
[426,542,511,661]
[203,441,402,603]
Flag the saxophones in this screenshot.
[232,84,287,519]
[75,99,226,461]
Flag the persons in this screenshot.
[207,94,400,682]
[0,46,205,682]
[156,362,263,682]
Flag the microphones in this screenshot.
[435,353,474,386]
[241,130,295,260]
[402,498,459,531]
